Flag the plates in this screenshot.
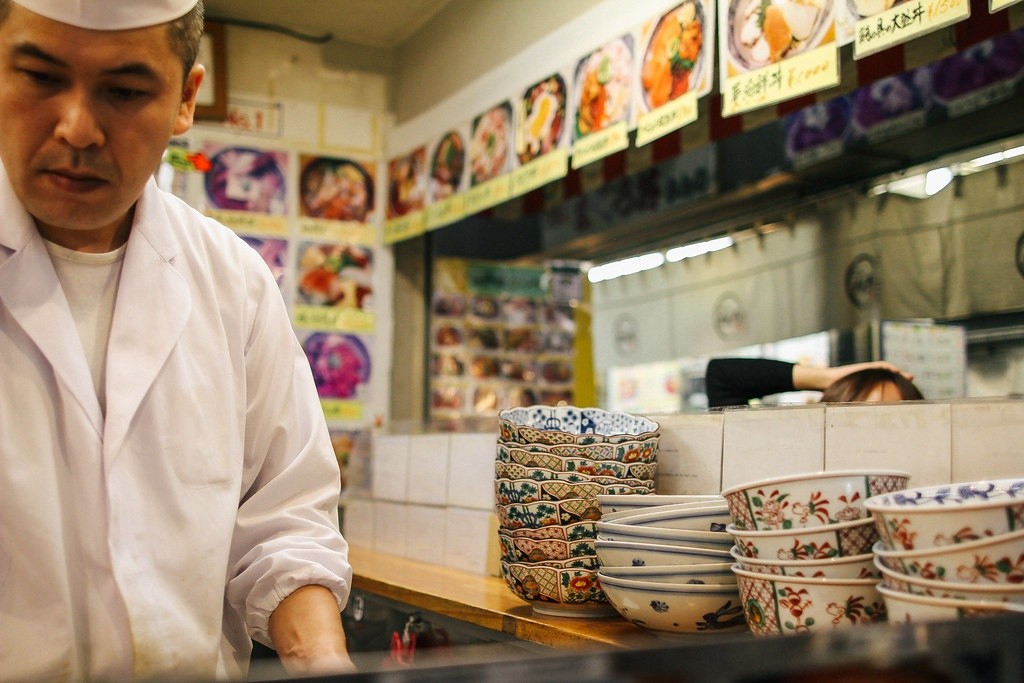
[296,241,373,308]
[302,330,372,402]
[386,1,706,222]
[297,155,373,225]
[204,145,286,218]
[236,236,284,288]
[727,0,836,71]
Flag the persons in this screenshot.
[703,357,925,410]
[0,0,360,683]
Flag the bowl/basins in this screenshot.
[729,561,888,638]
[876,581,1024,624]
[721,471,911,529]
[863,478,1024,550]
[874,555,1024,606]
[870,531,1024,585]
[724,517,882,560]
[729,546,882,580]
[493,404,749,646]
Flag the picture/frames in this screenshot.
[192,22,230,123]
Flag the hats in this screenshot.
[11,0,197,31]
